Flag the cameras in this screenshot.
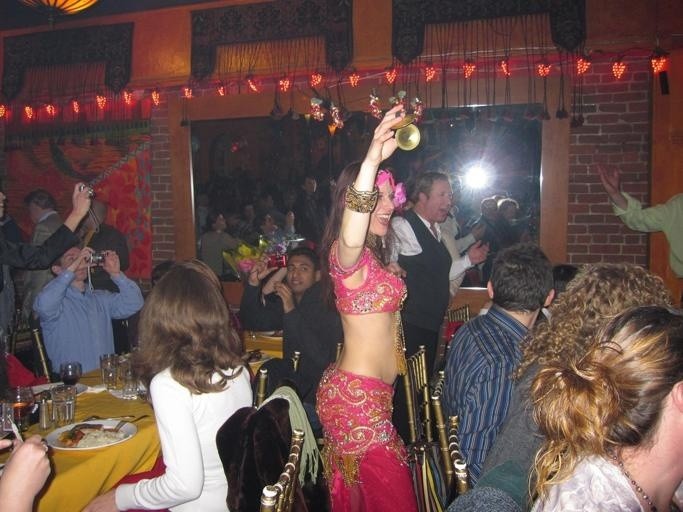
[80,184,96,199]
[90,252,107,266]
[271,255,286,267]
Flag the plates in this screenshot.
[259,330,283,341]
[43,419,138,453]
[241,348,273,366]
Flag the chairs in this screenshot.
[0,302,491,511]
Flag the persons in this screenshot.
[313,99,421,512]
[81,267,253,511]
[445,263,675,512]
[0,434,51,510]
[389,168,489,437]
[529,305,683,512]
[442,244,555,485]
[237,245,339,438]
[598,165,683,278]
[34,245,144,383]
[438,193,527,296]
[0,183,128,351]
[198,170,339,280]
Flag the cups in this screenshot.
[0,343,153,439]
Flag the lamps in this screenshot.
[16,0,100,30]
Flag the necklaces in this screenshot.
[608,449,657,512]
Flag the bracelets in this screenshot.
[340,183,380,215]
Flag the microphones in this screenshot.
[447,211,453,219]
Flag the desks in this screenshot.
[1,331,284,479]
